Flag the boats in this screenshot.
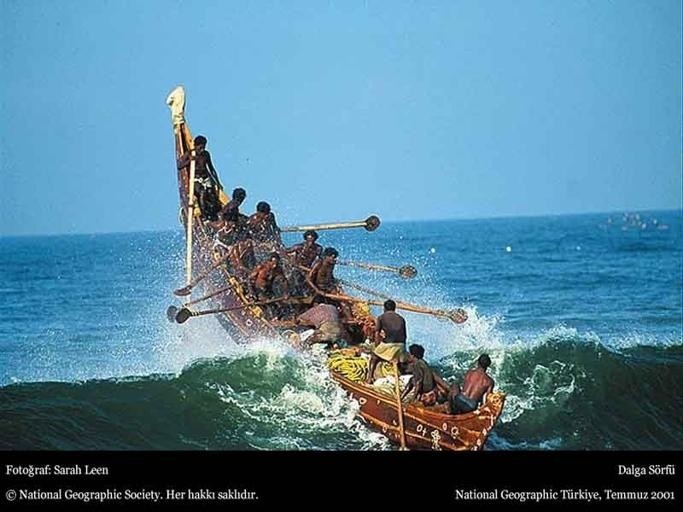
[165,86,504,452]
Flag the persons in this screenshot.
[175,134,494,417]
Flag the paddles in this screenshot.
[341,281,450,322]
[174,295,287,323]
[334,260,419,279]
[388,359,410,452]
[165,274,252,323]
[278,215,381,232]
[174,249,232,296]
[353,295,468,324]
